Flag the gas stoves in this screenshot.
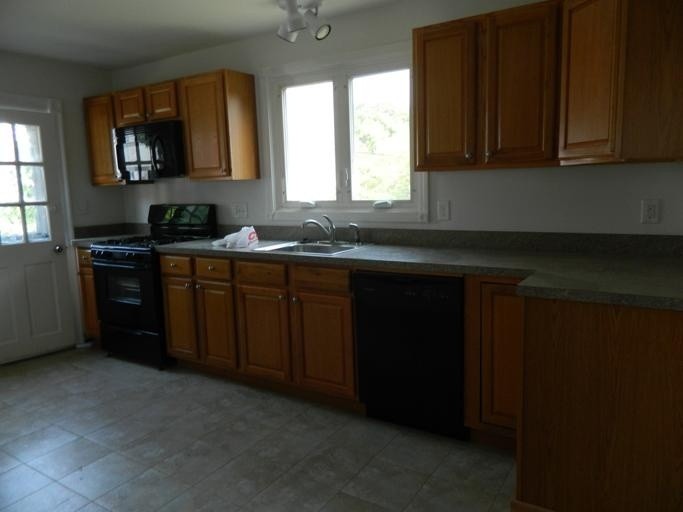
[88,235,207,261]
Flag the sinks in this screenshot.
[252,239,355,256]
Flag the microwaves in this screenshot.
[110,120,186,184]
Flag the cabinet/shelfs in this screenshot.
[406,1,558,172]
[77,248,100,339]
[553,0,681,167]
[232,259,369,420]
[81,90,155,187]
[458,275,522,448]
[109,78,179,127]
[178,68,260,183]
[160,254,236,387]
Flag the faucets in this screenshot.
[299,214,336,243]
[348,222,361,243]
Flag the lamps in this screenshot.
[273,0,331,45]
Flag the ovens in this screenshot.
[91,260,165,372]
